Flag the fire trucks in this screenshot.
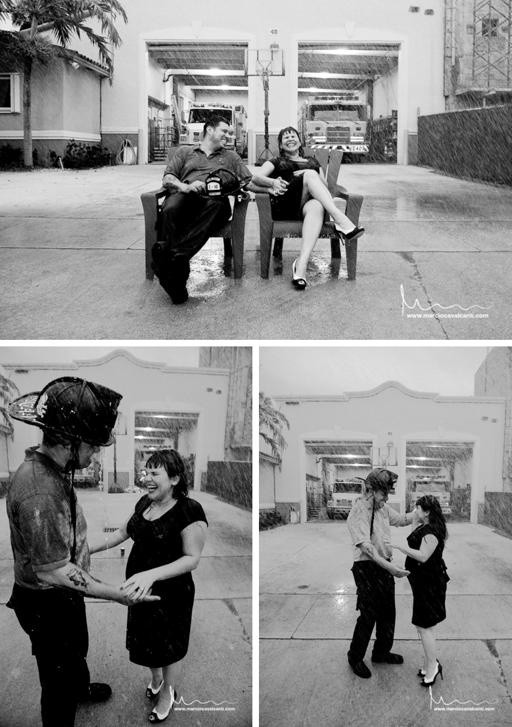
[70,456,101,488]
[409,477,453,522]
[325,478,365,519]
[137,442,174,488]
[297,95,370,163]
[178,100,248,158]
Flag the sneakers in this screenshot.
[346,659,372,678]
[89,682,112,703]
[371,652,404,664]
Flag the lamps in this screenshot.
[68,60,80,70]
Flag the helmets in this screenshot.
[9,377,123,447]
[355,467,398,494]
[205,168,250,202]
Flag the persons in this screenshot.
[5,376,162,727]
[383,495,451,687]
[151,115,286,305]
[88,449,208,723]
[347,468,416,679]
[252,127,365,290]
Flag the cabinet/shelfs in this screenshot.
[150,117,178,162]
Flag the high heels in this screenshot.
[146,679,164,699]
[417,658,443,687]
[334,223,365,245]
[292,257,307,289]
[148,685,177,724]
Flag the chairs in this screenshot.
[254,147,364,280]
[141,146,250,279]
